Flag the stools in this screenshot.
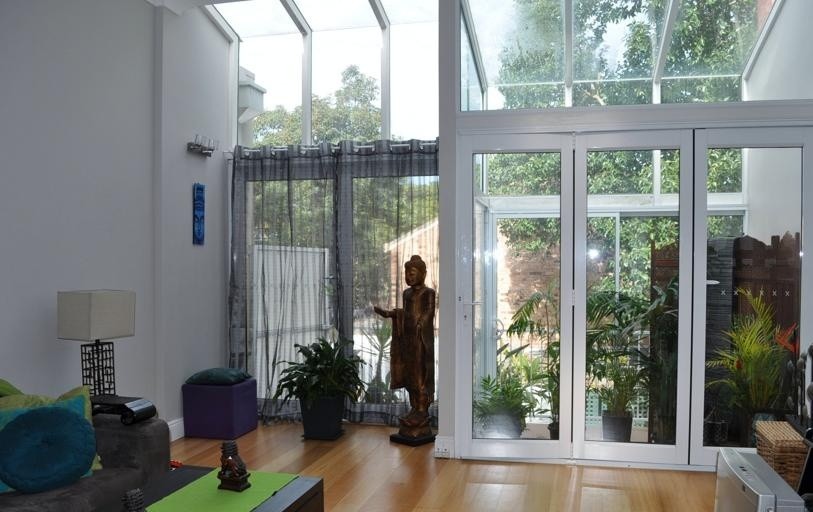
[181,378,257,440]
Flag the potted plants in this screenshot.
[705,287,798,447]
[271,336,370,441]
[472,289,661,442]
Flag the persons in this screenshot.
[373,254,435,429]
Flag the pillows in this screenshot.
[184,367,252,385]
[0,385,103,493]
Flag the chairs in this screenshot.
[0,377,169,512]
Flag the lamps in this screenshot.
[55,289,135,401]
